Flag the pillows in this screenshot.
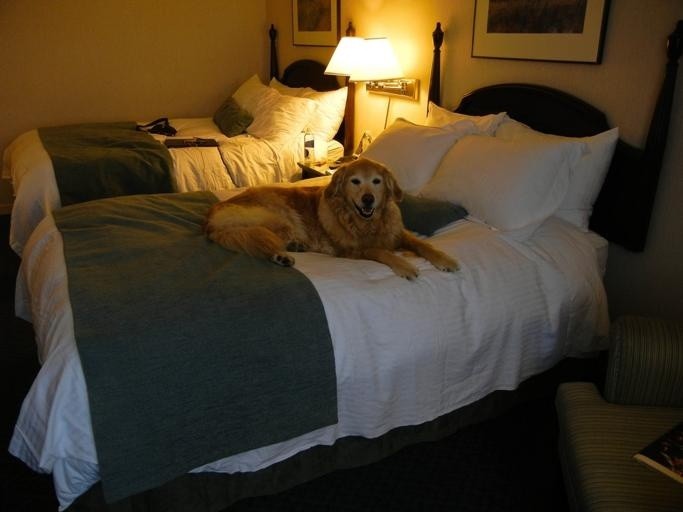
[423,134,588,242]
[211,72,349,140]
[422,101,510,137]
[359,115,475,195]
[392,188,469,238]
[495,117,628,232]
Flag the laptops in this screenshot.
[165,139,218,148]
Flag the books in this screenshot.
[632,418,683,489]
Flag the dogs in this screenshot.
[205,156,460,283]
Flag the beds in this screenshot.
[3,20,360,257]
[9,20,682,512]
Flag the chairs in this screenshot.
[553,313,682,511]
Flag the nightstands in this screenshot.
[296,152,358,178]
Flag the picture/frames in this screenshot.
[290,1,341,48]
[470,0,611,65]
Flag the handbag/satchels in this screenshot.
[134,117,178,138]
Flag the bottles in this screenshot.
[303,128,316,166]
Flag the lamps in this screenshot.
[319,34,420,101]
